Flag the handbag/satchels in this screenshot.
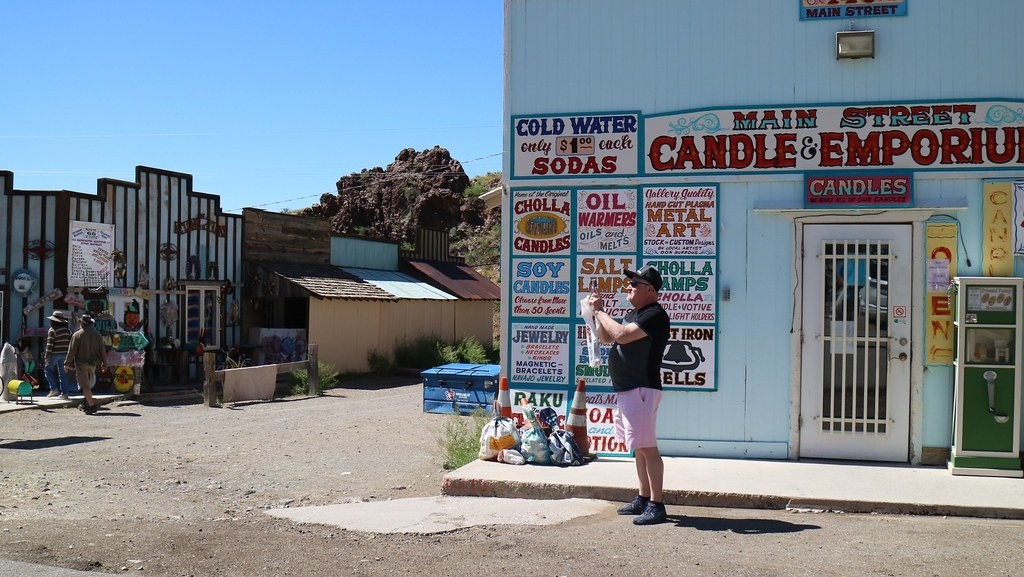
[580,295,601,368]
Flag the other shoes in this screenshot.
[47,389,59,397]
[58,393,68,400]
[78,401,89,412]
[85,405,97,415]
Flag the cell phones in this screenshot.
[591,279,597,294]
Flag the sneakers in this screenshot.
[617,496,649,515]
[633,502,667,525]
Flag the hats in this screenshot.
[77,314,95,324]
[623,267,663,293]
[46,311,68,323]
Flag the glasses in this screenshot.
[626,280,654,292]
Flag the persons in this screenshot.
[43,311,72,399]
[63,314,108,413]
[838,245,870,320]
[588,266,670,525]
